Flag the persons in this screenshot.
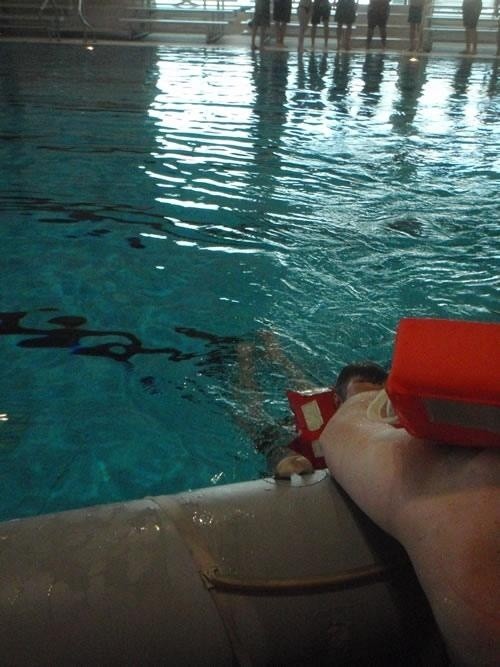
[251,59,499,101]
[317,386,498,665]
[233,328,391,478]
[248,0,486,59]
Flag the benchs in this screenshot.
[116,5,358,44]
